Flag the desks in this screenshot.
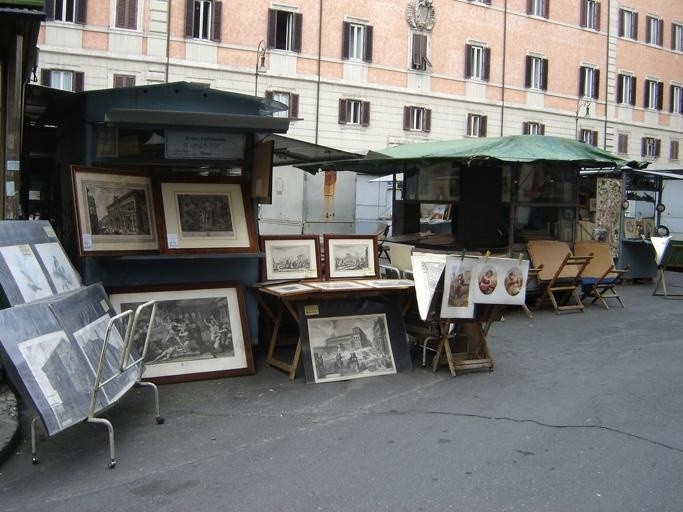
[244,279,415,381]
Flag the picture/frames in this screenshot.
[156,180,257,253]
[324,234,380,279]
[259,280,415,298]
[70,165,162,257]
[105,280,256,387]
[259,235,322,282]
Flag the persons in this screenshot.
[124,312,236,367]
[313,348,391,378]
[177,193,232,235]
[454,273,464,300]
[505,271,523,296]
[478,270,495,294]
